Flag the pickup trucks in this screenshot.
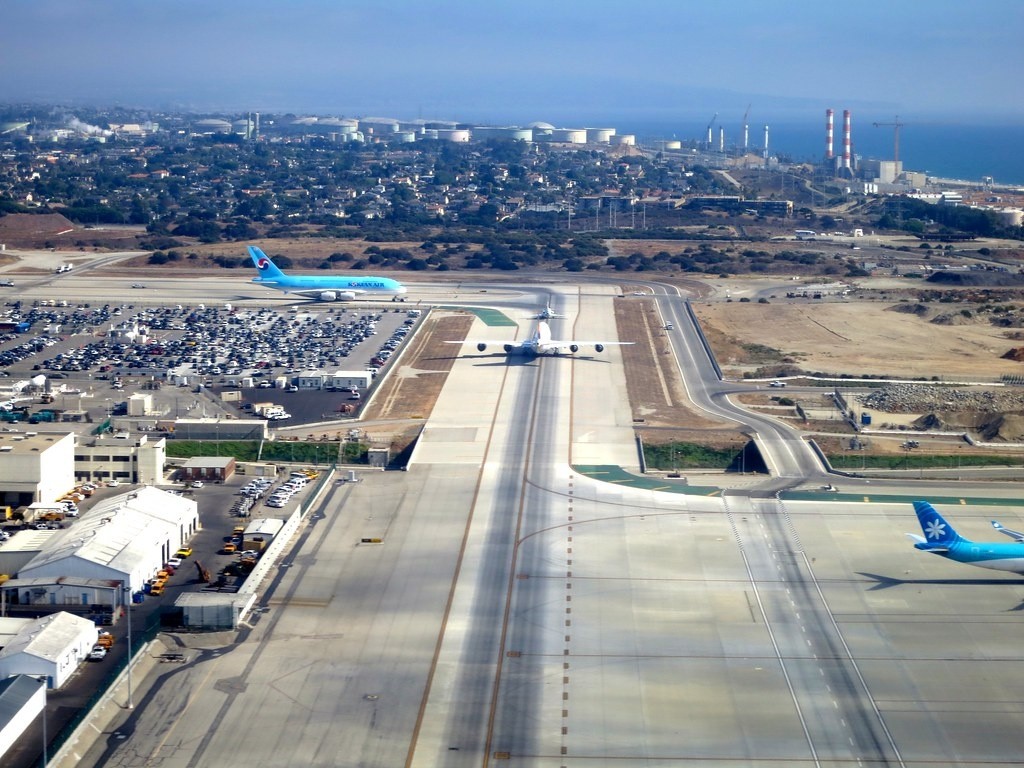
[771,381,786,387]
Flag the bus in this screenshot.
[795,230,816,240]
[849,228,863,238]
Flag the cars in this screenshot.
[0,262,148,291]
[0,300,420,661]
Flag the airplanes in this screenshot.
[243,245,409,302]
[442,301,638,360]
[905,500,1023,575]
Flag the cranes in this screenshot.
[872,114,941,182]
